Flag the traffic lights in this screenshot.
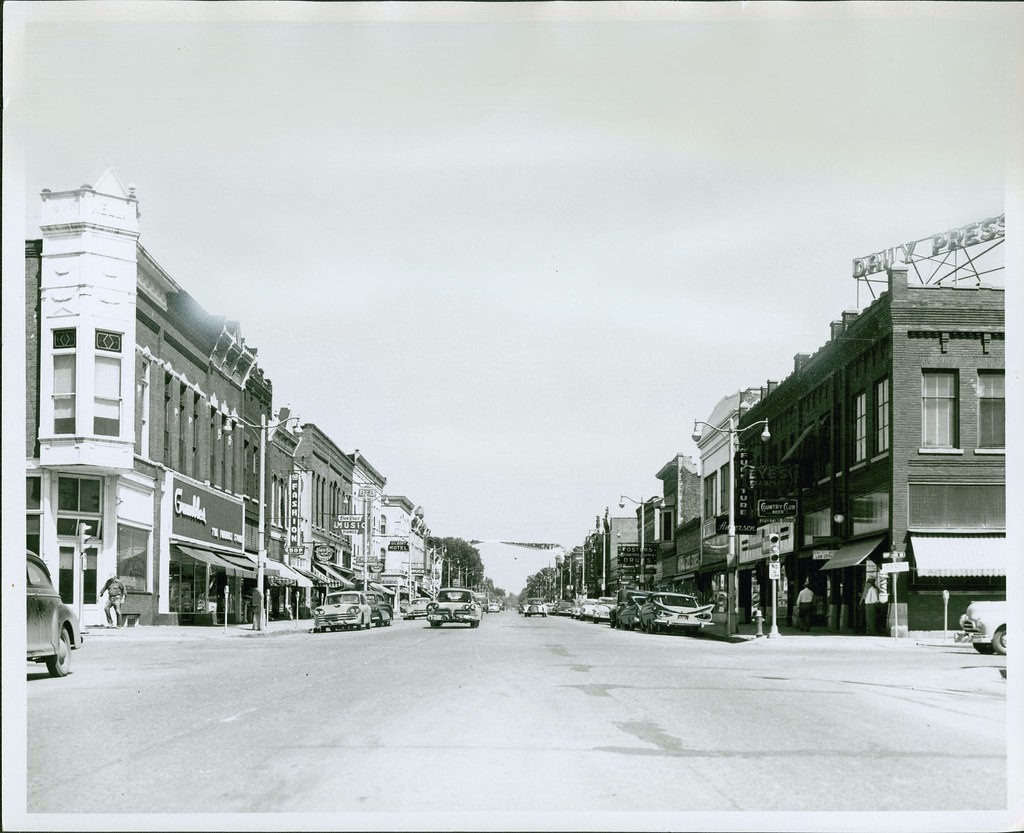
[79,523,93,554]
[769,532,781,563]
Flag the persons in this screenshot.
[311,592,319,618]
[863,577,880,636]
[796,582,814,632]
[98,571,127,628]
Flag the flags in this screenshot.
[333,514,370,535]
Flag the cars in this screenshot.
[404,598,431,620]
[475,596,490,614]
[358,591,393,628]
[952,597,1005,653]
[25,549,84,680]
[517,594,718,636]
[427,587,484,630]
[488,602,500,613]
[313,590,372,632]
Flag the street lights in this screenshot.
[691,417,771,639]
[618,495,665,589]
[222,412,302,629]
[570,546,596,595]
[424,544,505,605]
[392,534,424,605]
[342,490,389,591]
[588,529,620,597]
[527,555,585,602]
